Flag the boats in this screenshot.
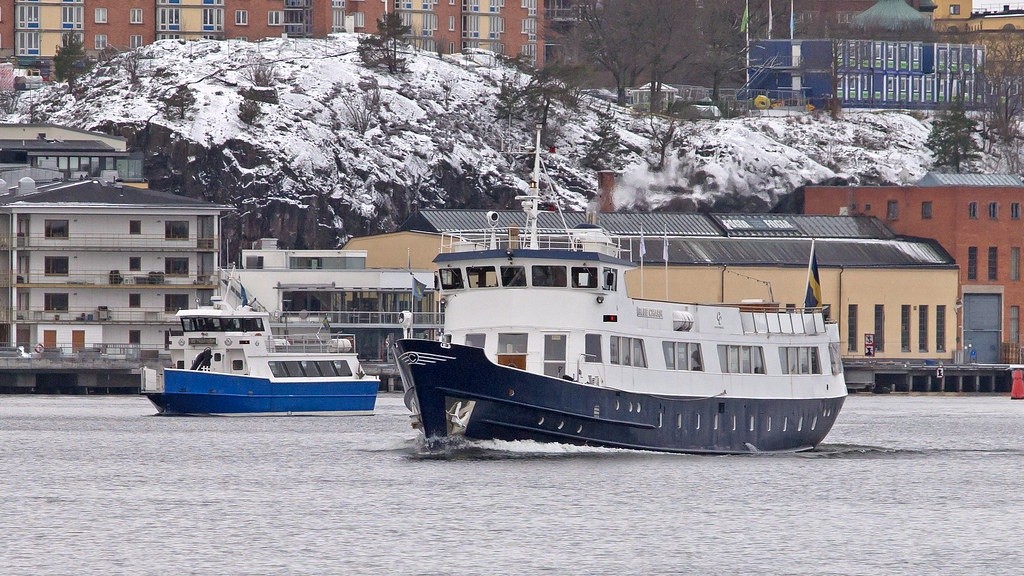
[140,264,382,419]
[388,124,850,455]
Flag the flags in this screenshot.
[768,0,773,33]
[789,0,795,33]
[740,4,749,35]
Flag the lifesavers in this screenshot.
[34,343,44,353]
